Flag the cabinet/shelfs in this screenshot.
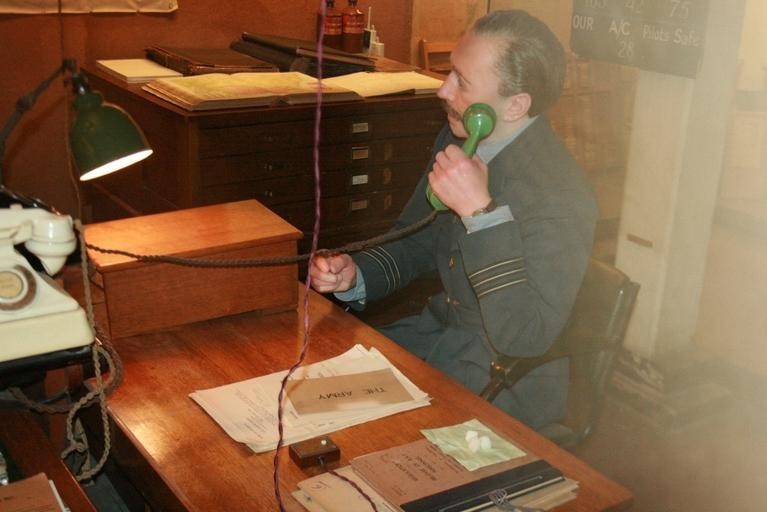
[80,55,452,315]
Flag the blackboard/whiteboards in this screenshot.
[568,0,710,78]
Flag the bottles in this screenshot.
[318,0,343,50]
[340,0,364,53]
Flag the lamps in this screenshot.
[0,56,154,183]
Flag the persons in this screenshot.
[308,10,598,428]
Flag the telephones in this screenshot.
[0,202,96,363]
[425,103,497,211]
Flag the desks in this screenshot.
[49,263,635,512]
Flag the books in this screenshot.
[0,472,66,512]
[290,419,580,512]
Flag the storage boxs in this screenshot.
[72,196,307,343]
